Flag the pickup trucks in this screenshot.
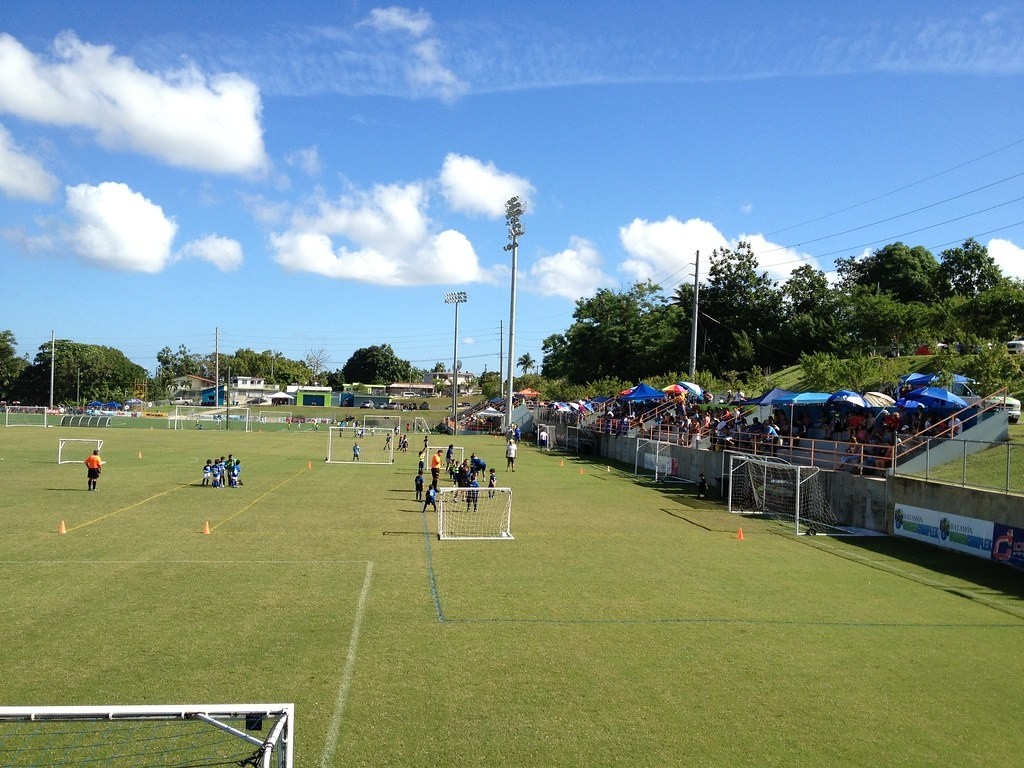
[171,397,193,406]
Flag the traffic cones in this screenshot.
[57,521,68,534]
[202,521,211,535]
[580,467,584,475]
[137,452,142,459]
[560,459,565,467]
[307,460,312,469]
[735,526,745,540]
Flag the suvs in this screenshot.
[948,380,1019,425]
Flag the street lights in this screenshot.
[443,290,469,435]
[498,194,530,434]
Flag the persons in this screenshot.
[202,460,212,487]
[466,475,479,512]
[697,473,709,499]
[220,456,227,487]
[195,417,204,430]
[338,394,975,487]
[453,459,470,503]
[422,484,438,513]
[286,418,320,431]
[229,459,241,488]
[84,449,103,491]
[414,471,424,501]
[212,459,222,488]
[225,454,243,486]
[488,468,496,498]
[2,405,86,414]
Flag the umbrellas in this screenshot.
[518,388,540,401]
[125,399,142,409]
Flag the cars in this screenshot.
[359,399,431,410]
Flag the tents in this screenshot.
[616,370,975,436]
[104,401,123,411]
[87,401,106,410]
